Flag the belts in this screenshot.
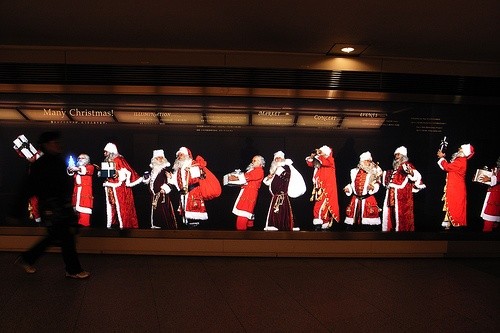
[353,194,370,200]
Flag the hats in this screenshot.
[104,142,119,157]
[274,150,284,160]
[176,147,193,160]
[394,146,408,158]
[360,150,372,162]
[461,144,474,160]
[320,144,333,158]
[153,149,165,158]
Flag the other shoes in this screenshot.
[14,260,35,274]
[66,271,90,278]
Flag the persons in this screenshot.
[232,156,265,231]
[166,147,208,229]
[14,132,90,279]
[437,143,474,230]
[472,159,500,232]
[378,146,426,231]
[263,151,293,231]
[93,143,143,228]
[67,154,94,228]
[306,145,340,232]
[28,194,42,226]
[143,149,178,230]
[343,151,382,231]
